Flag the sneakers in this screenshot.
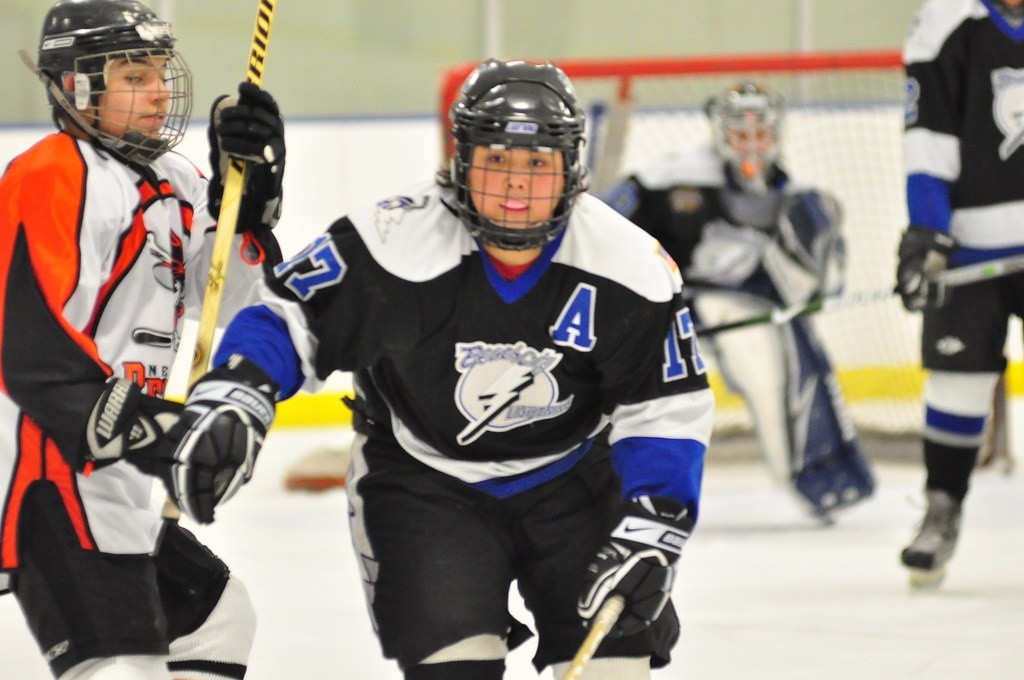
[902,491,961,592]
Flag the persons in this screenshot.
[604,81,878,529]
[892,1,1023,571]
[160,56,716,679]
[1,0,286,679]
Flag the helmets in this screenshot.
[443,63,588,252]
[17,1,192,166]
[702,82,783,178]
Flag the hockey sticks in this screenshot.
[685,241,1024,356]
[154,0,285,543]
[550,590,632,680]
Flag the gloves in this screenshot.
[205,83,287,233]
[163,351,278,526]
[80,375,190,486]
[895,226,955,311]
[575,495,696,638]
